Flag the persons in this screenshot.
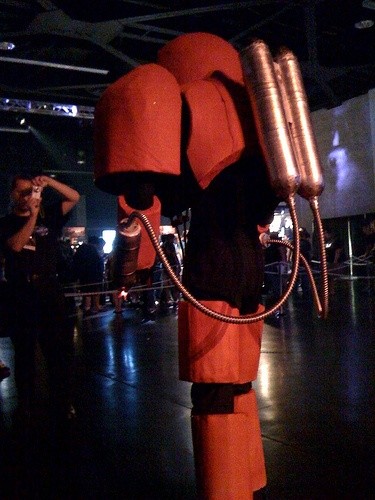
[263,227,344,305]
[95,33,269,500]
[0,168,81,428]
[58,234,181,326]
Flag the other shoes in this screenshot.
[79,304,126,316]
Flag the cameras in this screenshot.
[31,186,41,199]
